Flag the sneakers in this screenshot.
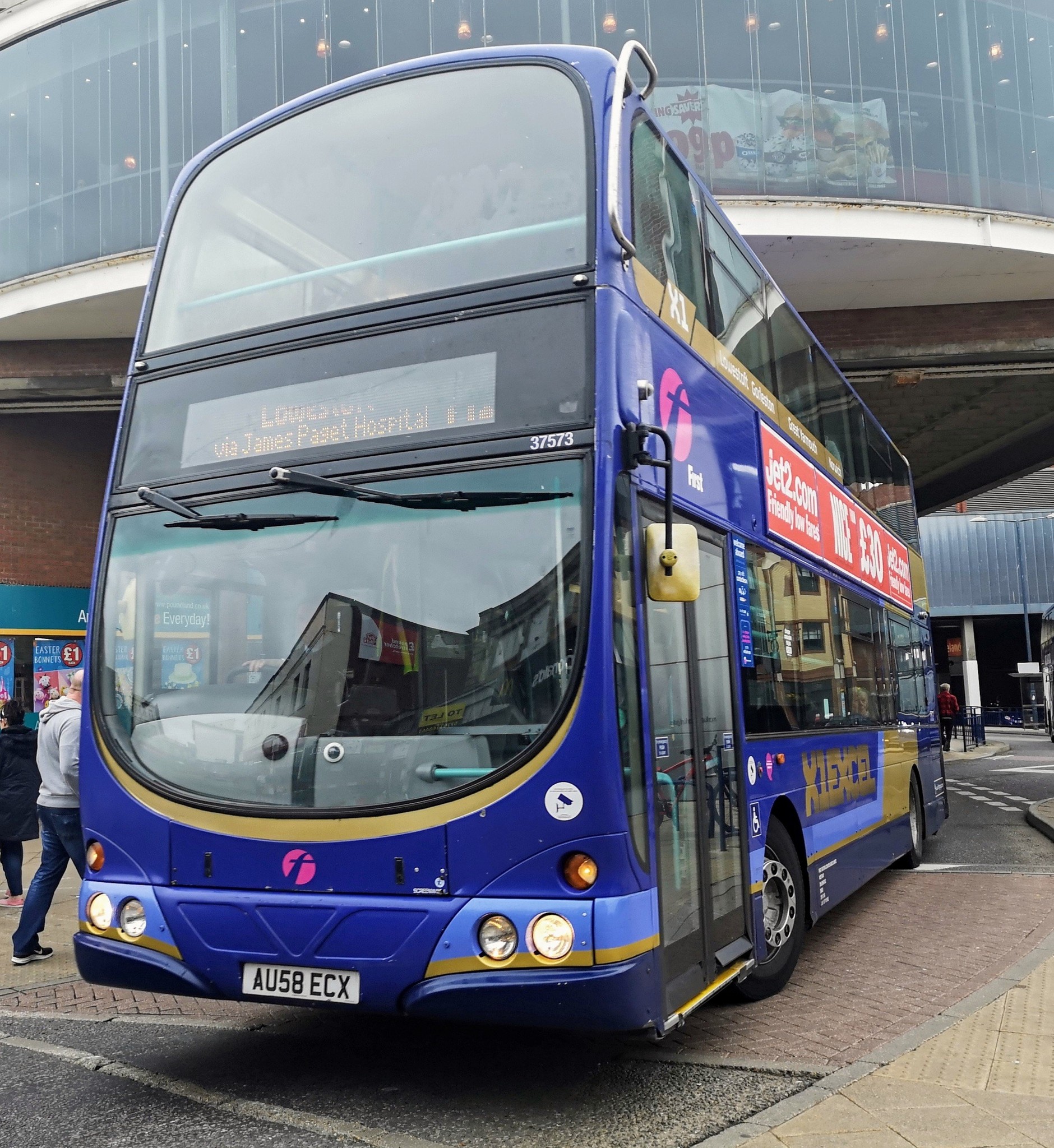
[5,889,11,898]
[11,945,53,964]
[0,895,24,907]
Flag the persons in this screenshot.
[852,687,877,719]
[0,700,43,908]
[242,561,353,672]
[938,683,959,751]
[11,668,84,964]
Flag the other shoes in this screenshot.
[943,741,950,751]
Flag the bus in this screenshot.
[70,37,949,1050]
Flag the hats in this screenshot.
[168,662,197,684]
